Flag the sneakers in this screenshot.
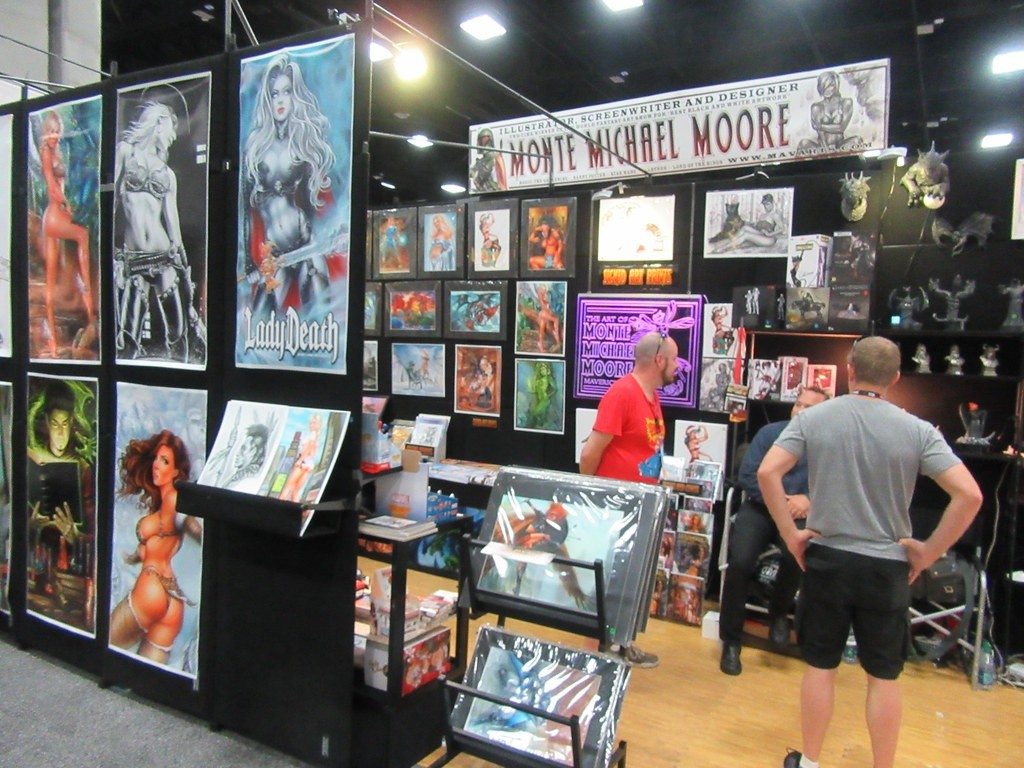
[611,642,659,668]
[769,614,790,646]
[721,641,741,675]
[784,748,802,768]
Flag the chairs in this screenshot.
[718,486,800,619]
[846,452,1010,693]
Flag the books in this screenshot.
[450,628,632,768]
[456,469,670,648]
[648,456,722,623]
[389,414,451,460]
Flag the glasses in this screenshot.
[655,333,667,361]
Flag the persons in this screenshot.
[580,332,679,667]
[711,305,728,338]
[685,547,704,576]
[757,337,984,768]
[719,387,831,676]
[660,542,668,558]
[684,514,706,535]
[684,424,713,463]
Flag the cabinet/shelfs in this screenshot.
[428,535,627,768]
[745,148,1024,462]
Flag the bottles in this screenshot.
[981,641,993,685]
